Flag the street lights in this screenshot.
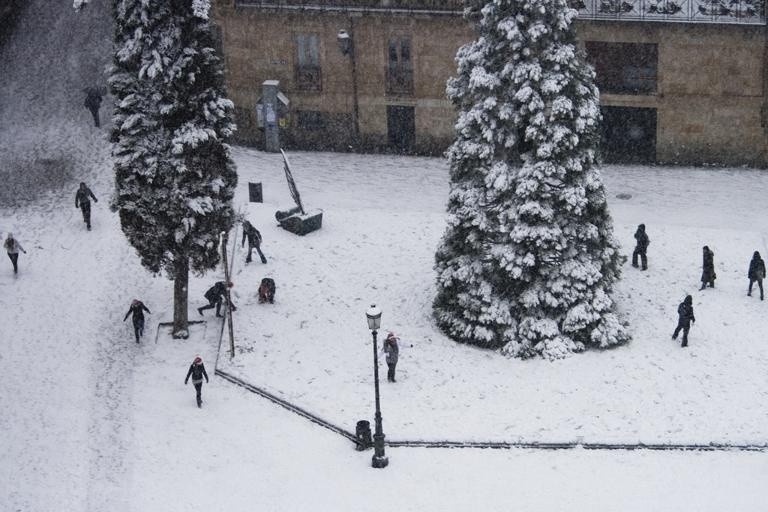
[365,302,389,468]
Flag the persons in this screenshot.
[671,295,696,348]
[384,332,401,382]
[699,245,716,291]
[75,181,98,231]
[630,223,651,271]
[240,220,269,266]
[196,282,237,319]
[3,231,28,278]
[257,276,276,306]
[746,250,766,301]
[122,299,151,345]
[83,87,105,129]
[184,356,210,409]
